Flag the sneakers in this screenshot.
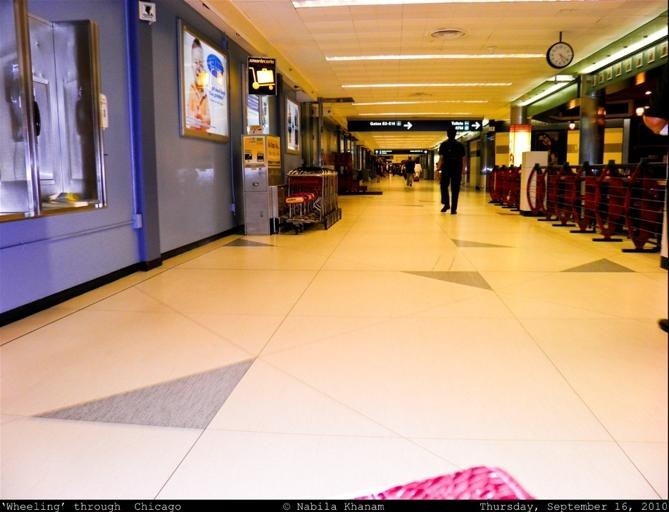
[657,318,668,333]
[440,204,458,214]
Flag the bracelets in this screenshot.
[437,170,439,171]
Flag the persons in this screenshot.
[385,155,422,186]
[642,69,668,334]
[435,127,465,214]
[188,39,211,132]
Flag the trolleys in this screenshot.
[283,166,342,234]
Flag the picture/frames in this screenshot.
[174,17,232,143]
[239,64,271,135]
[282,93,303,157]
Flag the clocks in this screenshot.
[547,31,574,69]
